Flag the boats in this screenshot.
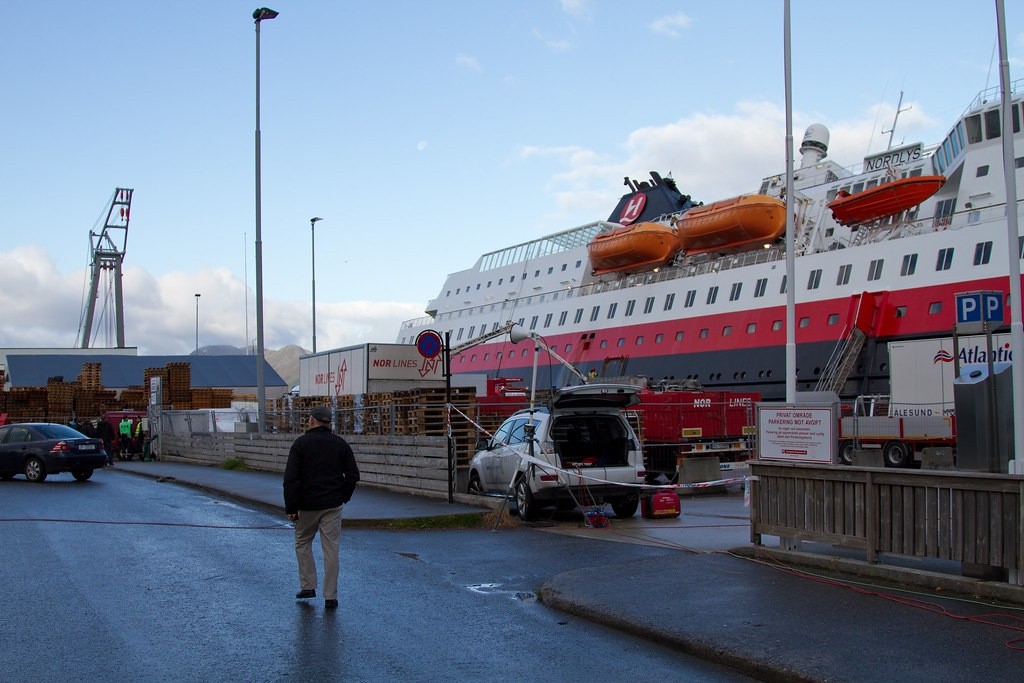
[393,71,1023,453]
[827,173,945,229]
[585,222,682,276]
[676,194,787,257]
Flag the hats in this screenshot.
[304,405,332,423]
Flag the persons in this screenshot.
[283,405,360,607]
[95,414,116,466]
[118,415,133,461]
[67,415,95,437]
[134,416,145,461]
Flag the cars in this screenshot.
[0,422,105,483]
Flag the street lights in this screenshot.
[310,216,324,355]
[249,6,281,440]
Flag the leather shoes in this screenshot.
[324,599,339,608]
[296,589,316,598]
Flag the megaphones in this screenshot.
[510,323,540,345]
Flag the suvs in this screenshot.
[468,383,646,524]
